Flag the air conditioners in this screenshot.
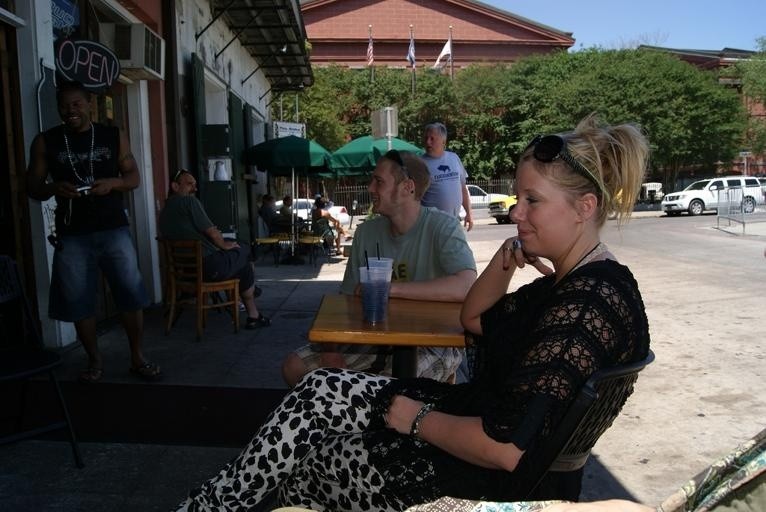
[97,21,166,81]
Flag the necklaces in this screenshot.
[63,120,95,185]
[562,241,601,281]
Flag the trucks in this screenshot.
[634,182,665,205]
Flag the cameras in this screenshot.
[74,185,92,196]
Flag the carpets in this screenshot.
[0,377,290,448]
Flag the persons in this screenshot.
[419,122,474,232]
[25,79,162,383]
[158,167,273,330]
[281,149,477,389]
[175,110,651,512]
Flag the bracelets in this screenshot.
[410,404,438,449]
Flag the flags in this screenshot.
[367,35,374,66]
[434,40,451,69]
[406,37,416,70]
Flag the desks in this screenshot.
[304,292,476,382]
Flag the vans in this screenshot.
[661,176,765,216]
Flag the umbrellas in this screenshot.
[240,135,427,256]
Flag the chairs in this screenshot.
[254,214,346,267]
[153,233,243,332]
[512,348,656,502]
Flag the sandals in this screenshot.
[245,312,271,331]
[253,287,262,297]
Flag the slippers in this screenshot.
[80,367,103,383]
[133,361,164,381]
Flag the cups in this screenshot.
[365,256,394,292]
[358,265,392,324]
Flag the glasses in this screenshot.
[524,134,602,193]
[386,149,409,179]
[173,168,187,180]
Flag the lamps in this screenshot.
[209,158,230,182]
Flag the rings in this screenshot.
[512,240,522,249]
[503,247,512,251]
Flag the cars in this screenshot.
[487,188,634,224]
[466,185,509,209]
[275,199,350,231]
[756,177,766,196]
